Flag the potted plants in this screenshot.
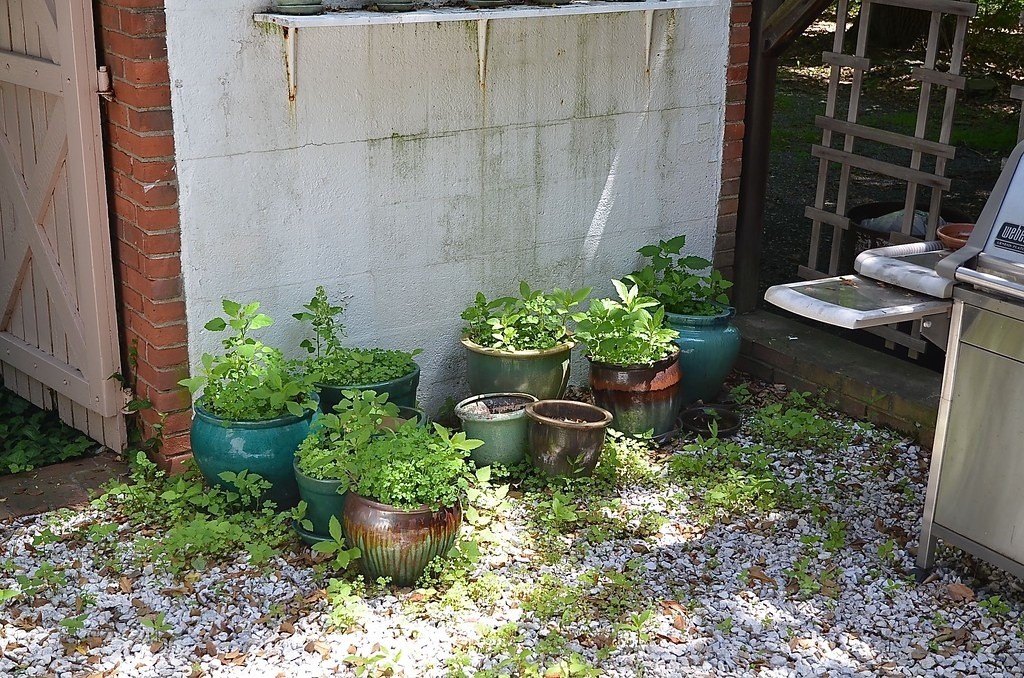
[178,286,483,587]
[460,235,742,443]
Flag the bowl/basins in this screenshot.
[936,224,975,248]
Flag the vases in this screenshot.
[526,400,613,480]
[455,393,539,469]
[844,202,974,268]
[937,224,975,248]
